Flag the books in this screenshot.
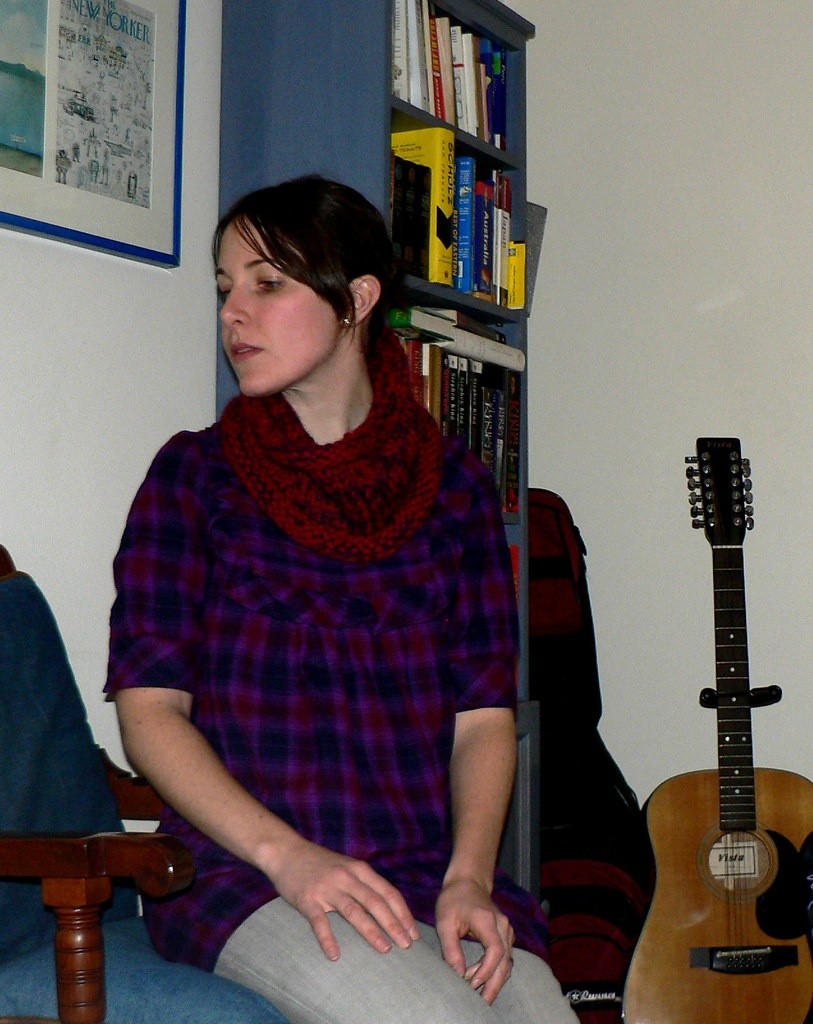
[392,0,506,151]
[390,127,525,310]
[389,301,526,525]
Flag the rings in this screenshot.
[509,956,514,965]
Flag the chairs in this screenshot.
[1,545,291,1024]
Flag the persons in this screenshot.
[101,172,579,1024]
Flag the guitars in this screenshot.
[619,435,813,1023]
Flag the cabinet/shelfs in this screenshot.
[217,0,532,707]
